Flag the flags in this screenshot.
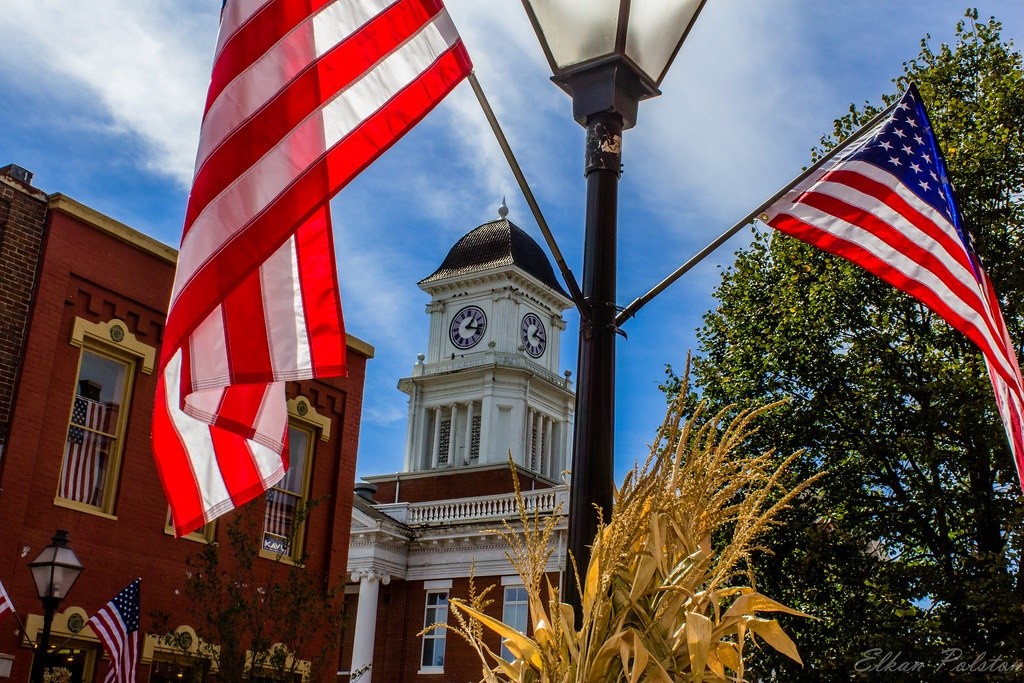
[149,0,475,541]
[264,466,298,537]
[84,577,141,683]
[755,81,1024,494]
[57,393,107,505]
[0,581,15,619]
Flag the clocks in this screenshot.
[521,313,547,359]
[449,305,487,350]
